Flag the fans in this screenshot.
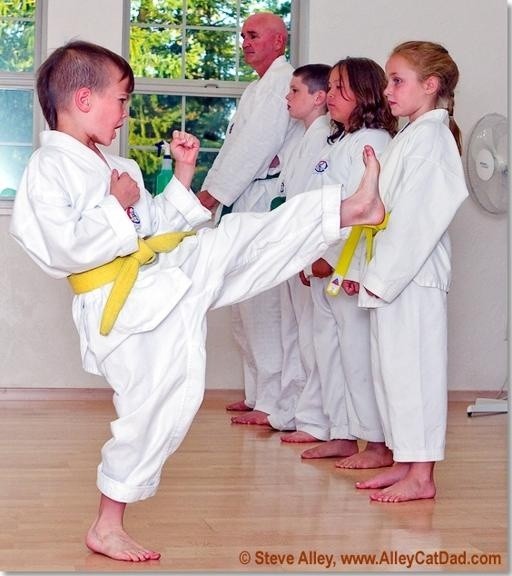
[462,110,511,418]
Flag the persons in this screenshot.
[301,57,398,468]
[8,41,385,562]
[195,13,295,424]
[324,41,469,504]
[257,63,339,444]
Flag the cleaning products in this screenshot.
[154,139,174,197]
